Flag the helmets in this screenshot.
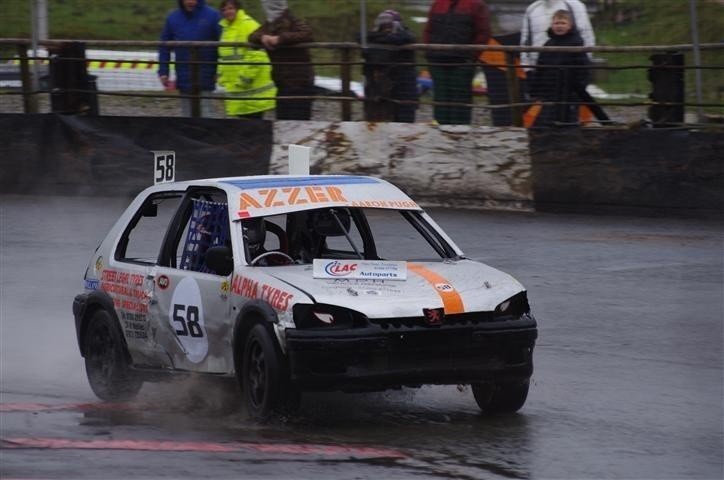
[219,207,266,253]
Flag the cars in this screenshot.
[73,172,538,420]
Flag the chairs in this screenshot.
[207,245,233,273]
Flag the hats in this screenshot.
[374,10,405,35]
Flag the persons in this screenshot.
[414,0,493,124]
[156,0,223,95]
[516,0,598,124]
[527,7,614,125]
[351,7,422,122]
[212,0,280,119]
[244,0,317,121]
[202,216,304,280]
